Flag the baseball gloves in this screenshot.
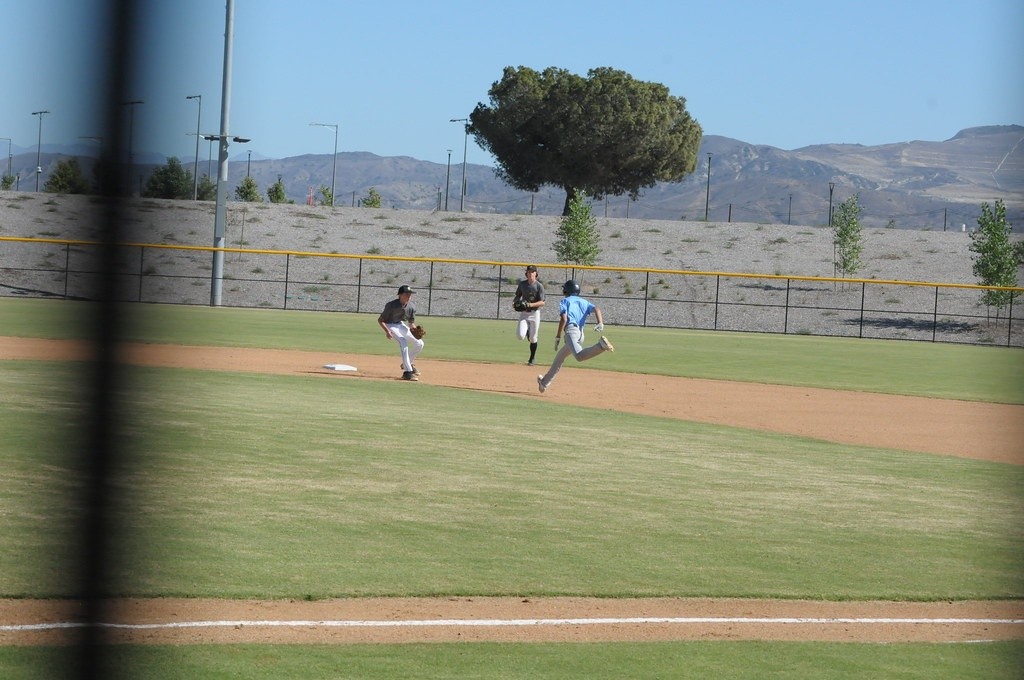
[410,325,426,340]
[513,299,531,313]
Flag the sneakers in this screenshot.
[538,375,546,393]
[402,371,418,381]
[527,328,530,342]
[400,364,421,376]
[599,336,615,353]
[528,358,534,365]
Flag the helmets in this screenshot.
[561,280,580,296]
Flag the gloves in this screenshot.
[593,323,604,333]
[555,336,560,351]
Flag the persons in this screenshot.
[535,280,613,392]
[378,285,424,382]
[512,265,545,368]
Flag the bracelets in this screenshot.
[555,336,561,338]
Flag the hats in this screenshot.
[397,285,418,295]
[525,265,537,272]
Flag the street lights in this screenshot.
[126,101,145,198]
[449,118,470,213]
[788,193,793,225]
[9,152,13,178]
[705,153,714,221]
[445,150,452,211]
[185,94,202,200]
[246,150,252,177]
[828,182,835,227]
[32,111,50,192]
[316,123,338,207]
